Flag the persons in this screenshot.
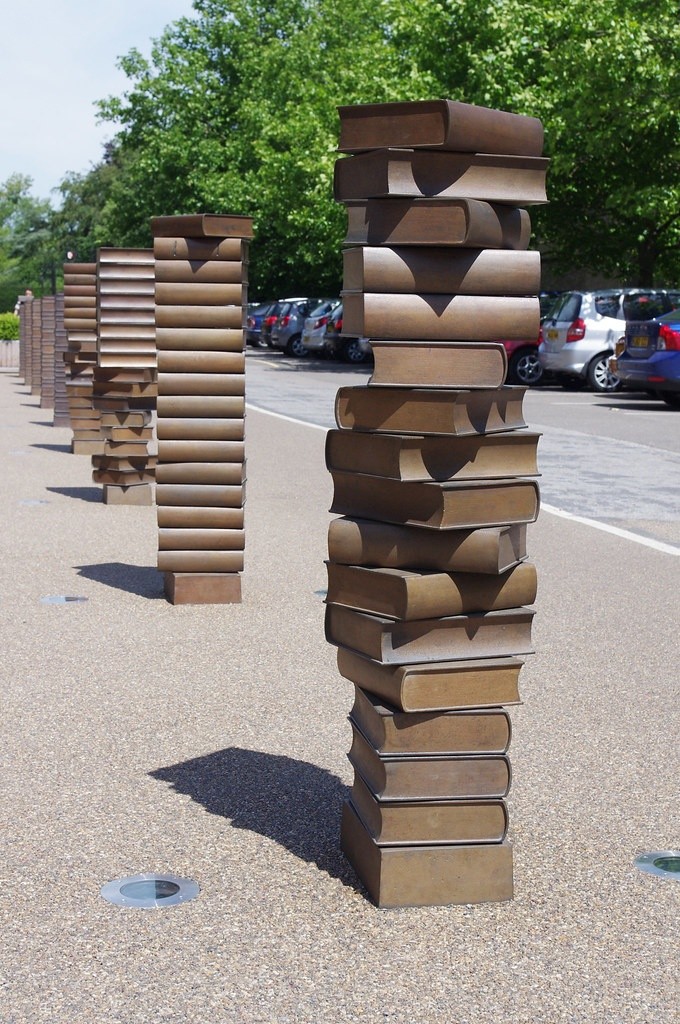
[13,288,33,316]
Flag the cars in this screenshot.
[498,288,679,403]
[248,297,375,363]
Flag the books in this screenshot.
[321,98,552,847]
[91,247,158,487]
[150,213,256,572]
[7,263,98,439]
